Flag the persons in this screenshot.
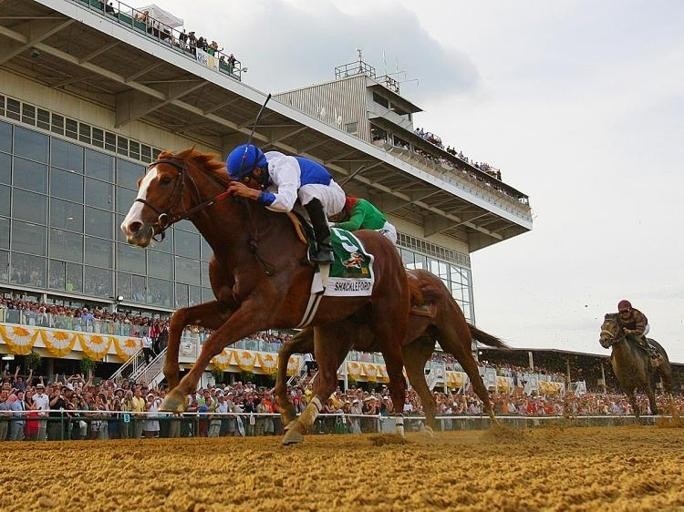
[329,192,401,247]
[370,118,528,207]
[224,143,347,267]
[98,0,239,72]
[2,293,684,442]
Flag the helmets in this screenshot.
[226,144,267,180]
[618,301,631,311]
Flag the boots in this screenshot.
[300,236,335,262]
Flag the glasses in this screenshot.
[242,145,258,182]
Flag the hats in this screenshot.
[36,384,44,389]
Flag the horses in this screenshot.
[274,269,512,437]
[120,143,410,445]
[599,313,672,417]
